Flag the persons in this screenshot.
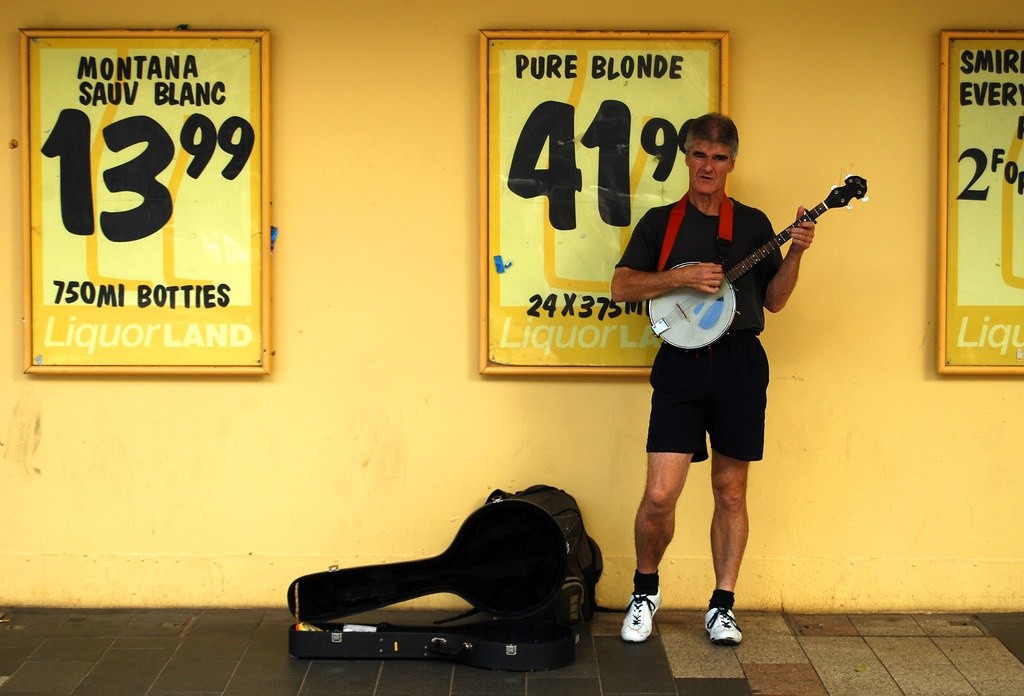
[611,112,816,645]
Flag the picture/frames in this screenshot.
[474,32,730,378]
[17,26,271,378]
[937,34,1024,378]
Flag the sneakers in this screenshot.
[621,587,661,642]
[704,606,743,646]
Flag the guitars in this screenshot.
[645,171,873,353]
